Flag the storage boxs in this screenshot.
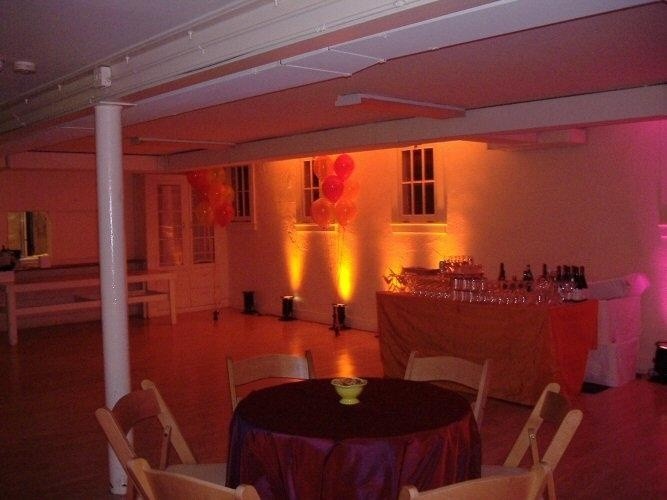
[596,296,641,342]
[584,336,640,388]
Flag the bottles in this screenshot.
[580,265,589,300]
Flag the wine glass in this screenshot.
[378,252,583,306]
[331,377,368,405]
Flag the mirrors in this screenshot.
[6,210,49,263]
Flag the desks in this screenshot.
[6,268,177,346]
[375,290,599,407]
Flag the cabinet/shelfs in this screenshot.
[132,174,233,319]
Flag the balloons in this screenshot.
[188,166,240,230]
[307,152,359,230]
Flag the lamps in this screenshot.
[279,295,297,322]
[241,290,257,315]
[647,342,667,386]
[329,303,351,331]
